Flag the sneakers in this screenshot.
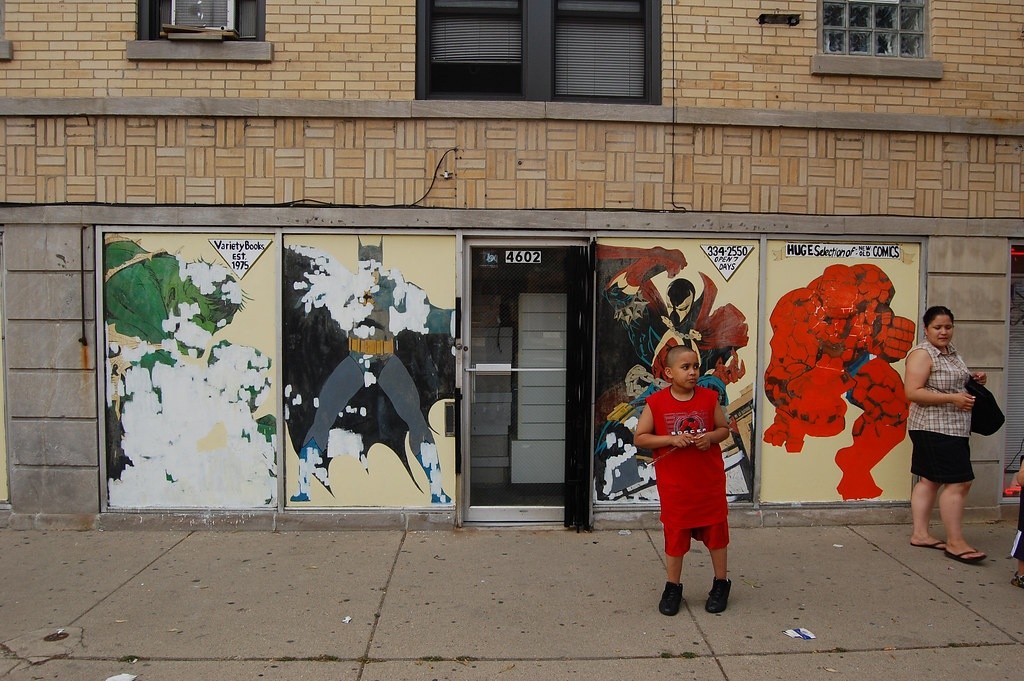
[659,582,683,615]
[705,577,731,612]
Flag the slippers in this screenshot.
[911,541,946,550]
[945,549,986,562]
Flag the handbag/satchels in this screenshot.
[965,376,1005,437]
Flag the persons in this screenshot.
[1009,455,1024,588]
[634,344,732,616]
[903,306,989,564]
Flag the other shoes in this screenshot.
[1011,571,1024,589]
